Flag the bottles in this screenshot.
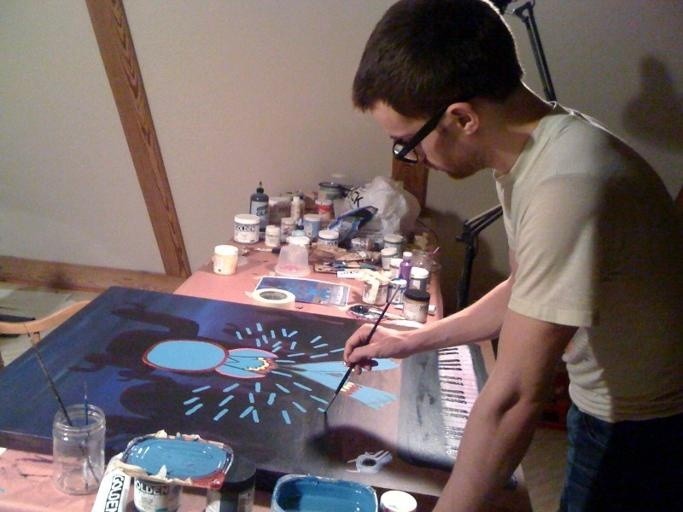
[233,180,342,249]
[399,252,414,280]
[363,234,431,324]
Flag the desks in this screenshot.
[0,210,536,512]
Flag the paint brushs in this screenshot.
[23,323,101,491]
[323,284,401,415]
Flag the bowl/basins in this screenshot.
[274,243,311,279]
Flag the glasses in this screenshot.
[393,113,444,163]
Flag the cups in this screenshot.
[52,405,106,496]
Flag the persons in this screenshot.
[342,0,683,511]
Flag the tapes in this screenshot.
[251,287,296,311]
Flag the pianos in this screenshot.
[397,345,518,486]
